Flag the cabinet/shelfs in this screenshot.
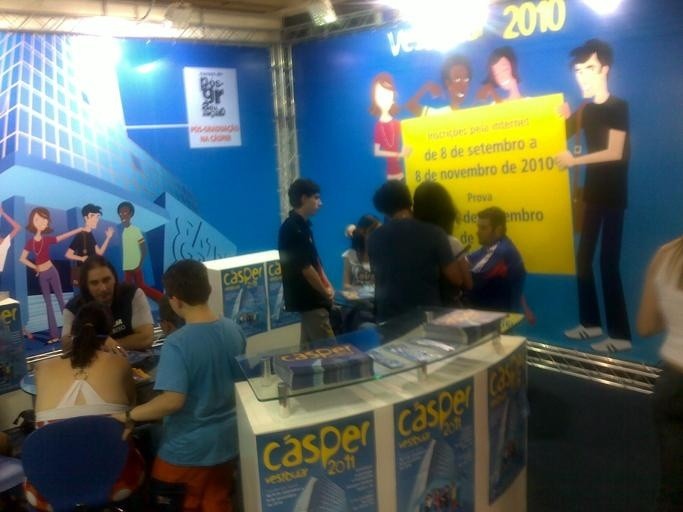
[231,305,529,512]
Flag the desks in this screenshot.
[16,344,161,397]
[330,291,379,328]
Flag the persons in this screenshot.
[636,235,682,511]
[279,180,526,351]
[24,255,252,511]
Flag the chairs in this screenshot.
[19,413,128,512]
